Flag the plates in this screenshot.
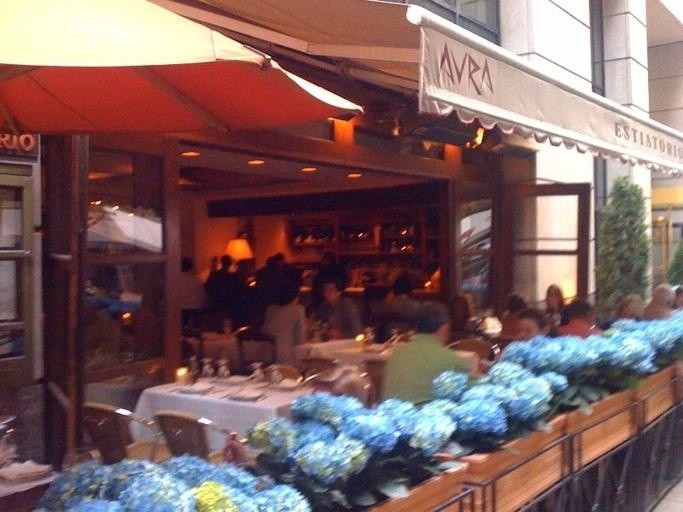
[0,460,53,484]
[184,382,212,392]
[234,389,262,401]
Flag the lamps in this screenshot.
[225,238,255,260]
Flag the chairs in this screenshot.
[32,310,482,471]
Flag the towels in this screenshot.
[1,459,52,482]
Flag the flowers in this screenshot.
[39,360,575,512]
[498,311,683,385]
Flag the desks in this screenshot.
[1,463,61,512]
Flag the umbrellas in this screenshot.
[0,0,365,133]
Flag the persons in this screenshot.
[180,254,349,334]
[263,281,362,362]
[379,278,421,337]
[451,286,600,340]
[600,283,682,330]
[381,303,475,405]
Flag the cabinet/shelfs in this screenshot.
[284,202,443,270]
[0,162,35,394]
